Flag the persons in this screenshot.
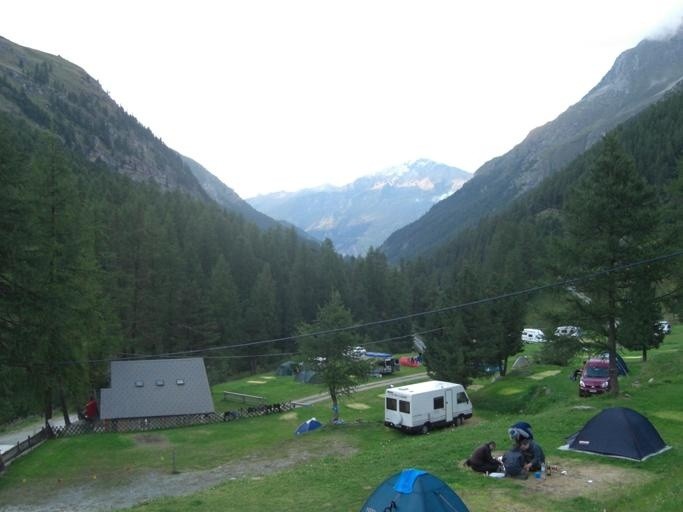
[517,438,544,472]
[568,366,583,380]
[468,441,500,473]
[500,443,527,479]
[507,420,533,443]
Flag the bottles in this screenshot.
[533,461,557,483]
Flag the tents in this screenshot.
[276,357,328,383]
[294,418,321,436]
[599,349,627,376]
[359,468,469,511]
[563,406,666,460]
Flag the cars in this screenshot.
[578,358,610,398]
[353,346,367,353]
[657,321,671,334]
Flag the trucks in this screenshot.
[521,329,550,344]
[382,380,473,434]
[554,325,580,337]
